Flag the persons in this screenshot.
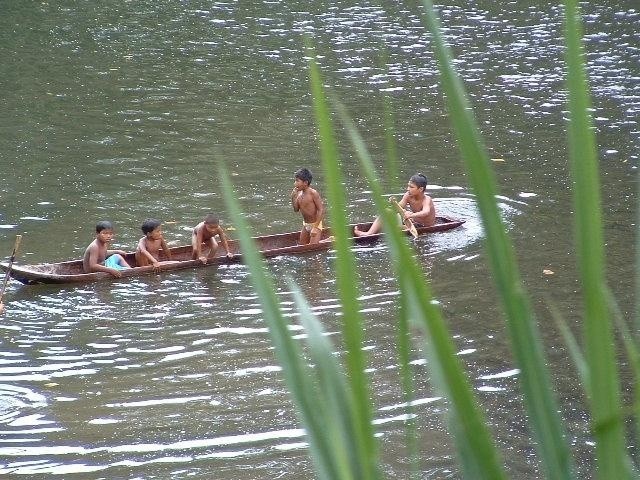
[354,173,435,236]
[82,220,132,278]
[190,212,233,265]
[134,218,171,274]
[291,168,324,244]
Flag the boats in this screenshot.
[0,213,466,287]
